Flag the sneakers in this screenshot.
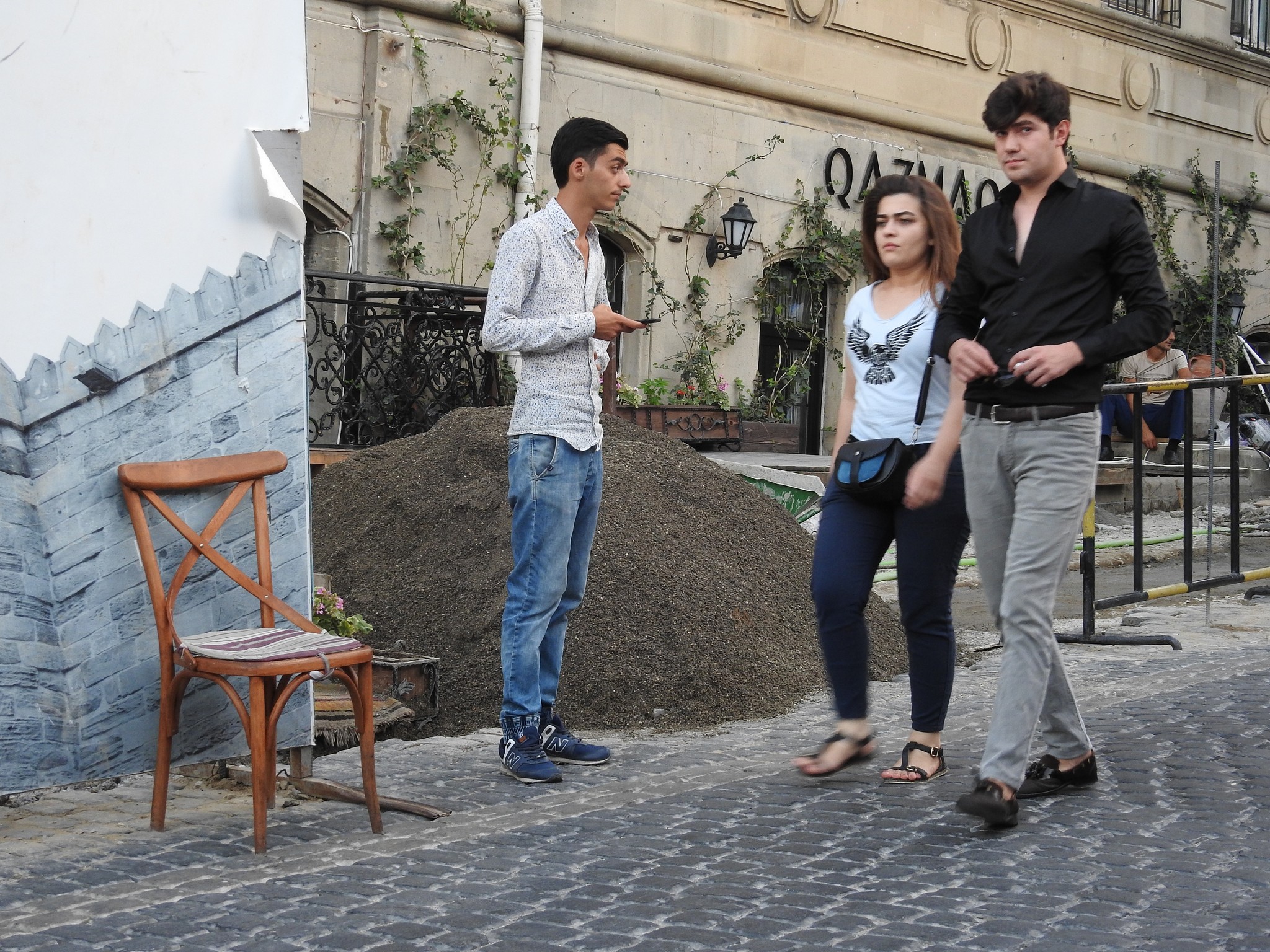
[538,714,611,765]
[498,726,564,783]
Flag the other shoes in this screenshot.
[1015,749,1098,798]
[957,779,1019,826]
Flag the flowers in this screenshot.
[599,371,731,411]
[311,585,374,639]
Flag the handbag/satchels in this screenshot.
[834,437,912,503]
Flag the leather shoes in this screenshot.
[1163,450,1182,465]
[1099,446,1114,461]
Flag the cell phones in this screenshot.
[633,318,661,324]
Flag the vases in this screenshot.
[1189,354,1229,438]
[616,405,743,451]
[330,648,439,720]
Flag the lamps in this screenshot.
[1228,293,1247,327]
[706,197,757,268]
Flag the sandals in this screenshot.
[881,741,948,783]
[793,733,876,777]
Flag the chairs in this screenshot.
[118,450,384,856]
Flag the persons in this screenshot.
[481,116,647,785]
[1097,314,1190,466]
[940,72,1174,828]
[792,173,985,784]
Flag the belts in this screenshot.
[965,401,1095,424]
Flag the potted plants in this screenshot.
[683,135,871,453]
